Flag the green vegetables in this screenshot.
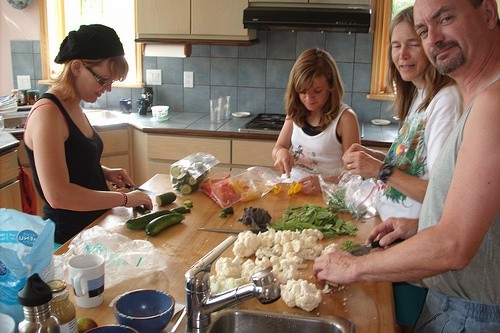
[275,188,367,239]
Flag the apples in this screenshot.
[75,317,97,333]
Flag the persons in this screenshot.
[312,0,500,333]
[24,24,152,253]
[342,6,463,222]
[272,48,360,194]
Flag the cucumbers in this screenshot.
[126,191,192,236]
[169,165,206,193]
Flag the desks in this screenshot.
[50,173,399,333]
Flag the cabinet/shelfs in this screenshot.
[0,127,276,217]
[132,0,258,45]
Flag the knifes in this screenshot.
[198,227,268,234]
[113,183,155,195]
[349,238,404,256]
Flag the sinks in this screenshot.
[169,307,356,333]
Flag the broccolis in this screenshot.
[208,227,338,312]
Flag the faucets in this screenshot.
[184,235,282,333]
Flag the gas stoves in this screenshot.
[238,111,286,134]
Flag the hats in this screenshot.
[54,24,123,64]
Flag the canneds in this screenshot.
[15,89,39,106]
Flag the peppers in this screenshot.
[202,173,303,208]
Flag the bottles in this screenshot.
[11,88,39,106]
[17,273,60,333]
[45,279,79,333]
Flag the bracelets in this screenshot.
[376,163,394,183]
[119,191,127,206]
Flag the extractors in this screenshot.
[243,0,375,34]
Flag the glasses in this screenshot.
[84,63,114,88]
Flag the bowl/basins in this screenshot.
[83,325,140,333]
[112,289,175,333]
[151,105,170,119]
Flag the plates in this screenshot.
[370,119,391,125]
[231,112,250,117]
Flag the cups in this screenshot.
[136,98,148,115]
[0,312,16,333]
[66,253,105,309]
[209,95,230,122]
[142,87,153,111]
